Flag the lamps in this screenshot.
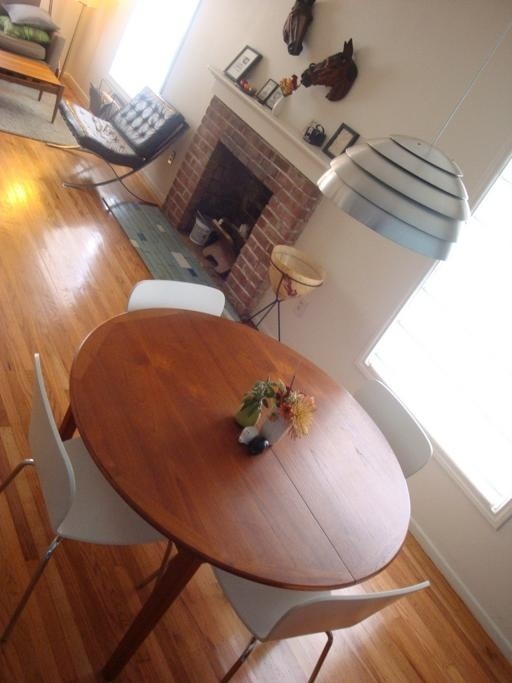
[316,21,511,260]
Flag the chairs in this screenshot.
[1,277,437,681]
[0,0,66,75]
[49,84,191,208]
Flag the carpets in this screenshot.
[0,77,78,147]
[103,195,216,288]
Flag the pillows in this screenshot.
[0,3,61,46]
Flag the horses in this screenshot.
[299,38,358,103]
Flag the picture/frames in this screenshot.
[257,79,280,103]
[224,45,262,82]
[323,123,360,159]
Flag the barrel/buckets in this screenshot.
[189,216,211,246]
